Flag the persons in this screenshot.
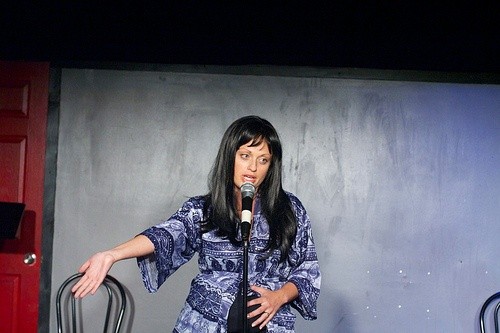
[72,115,321,333]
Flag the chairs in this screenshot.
[57,272,128,333]
[478,292,500,333]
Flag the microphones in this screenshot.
[240,181,256,239]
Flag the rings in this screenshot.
[264,312,270,316]
